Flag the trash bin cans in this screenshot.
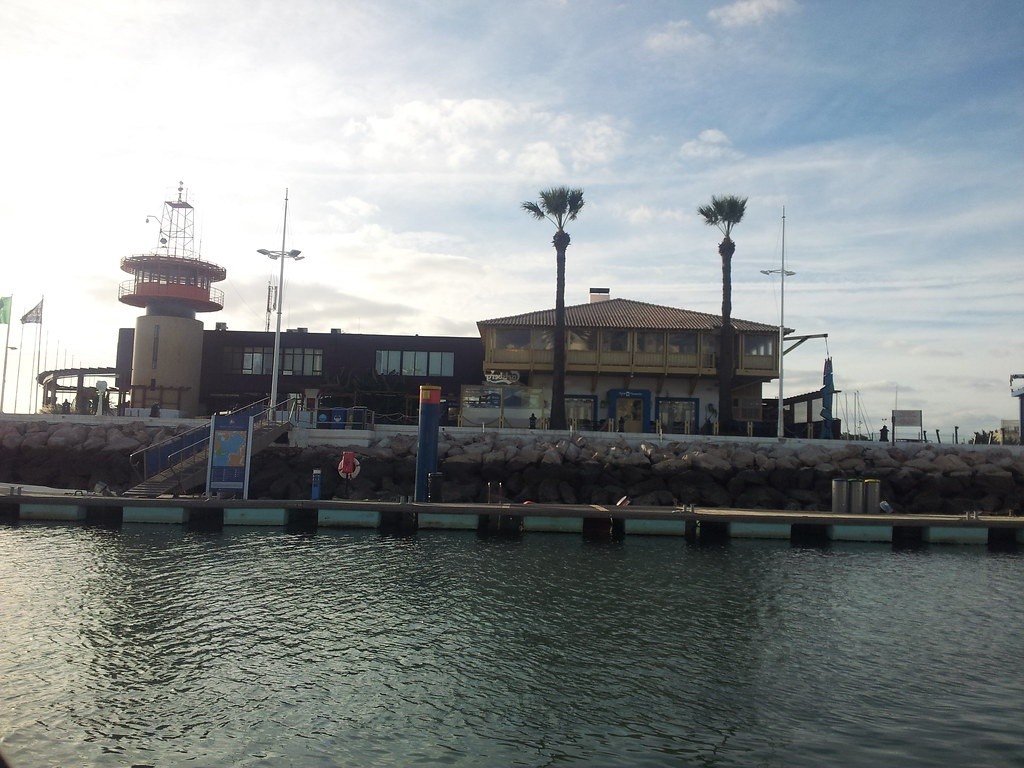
[831,477,882,515]
[316,407,352,430]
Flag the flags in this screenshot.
[21,300,42,324]
[0,297,11,324]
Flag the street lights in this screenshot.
[0,346,18,413]
[762,268,796,437]
[257,248,305,422]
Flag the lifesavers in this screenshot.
[337,457,361,480]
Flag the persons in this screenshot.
[150,402,160,417]
